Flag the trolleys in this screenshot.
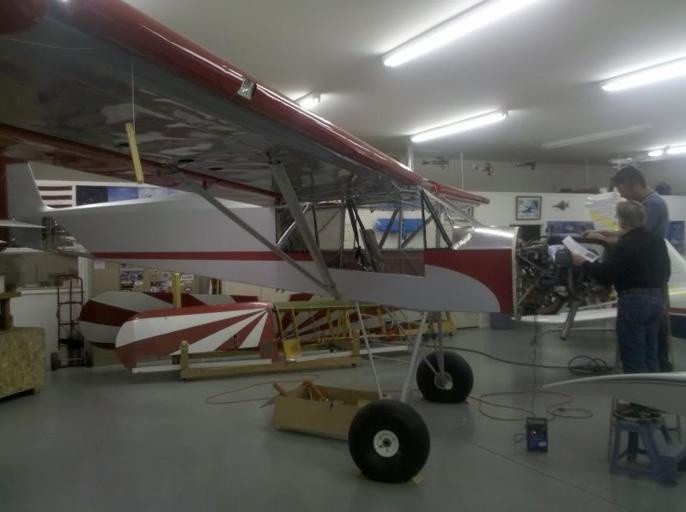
[50,273,94,370]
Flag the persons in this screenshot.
[571,200,672,419]
[581,164,668,239]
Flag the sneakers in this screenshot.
[614,405,663,421]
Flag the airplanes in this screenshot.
[0,0,686,484]
